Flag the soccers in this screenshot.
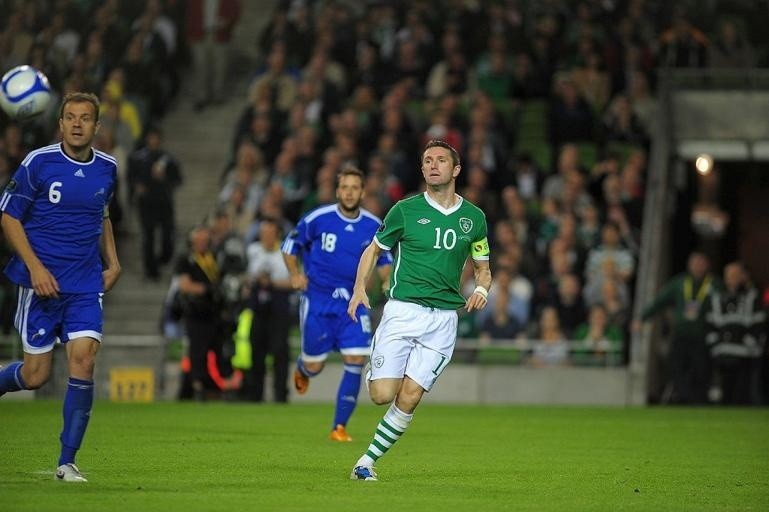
[0,64,50,120]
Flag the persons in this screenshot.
[0,92,122,483]
[280,167,394,442]
[346,139,492,481]
[1,1,246,336]
[163,0,768,403]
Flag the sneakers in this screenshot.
[54,462,87,482]
[329,424,350,441]
[351,465,378,481]
[294,369,309,394]
